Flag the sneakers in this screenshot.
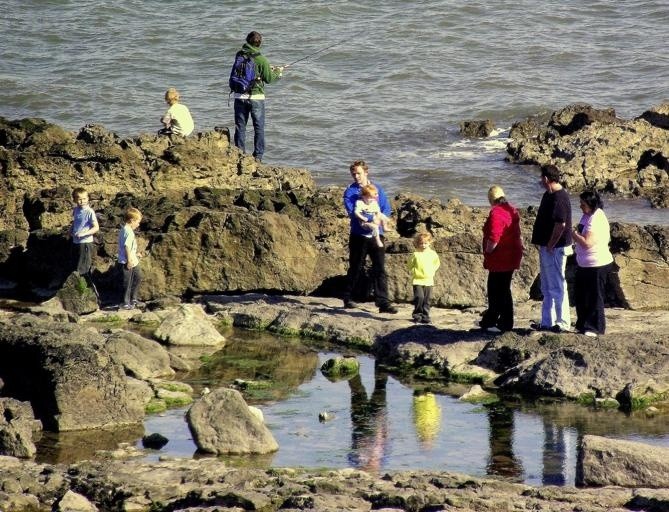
[343,299,358,308]
[379,303,398,314]
[531,323,598,337]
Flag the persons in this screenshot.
[342,161,399,313]
[410,389,443,452]
[485,405,524,485]
[479,185,522,332]
[531,163,573,333]
[355,186,394,248]
[229,31,283,162]
[158,88,194,137]
[66,187,100,277]
[408,234,440,324]
[572,187,614,336]
[119,207,142,310]
[539,420,565,485]
[344,354,392,475]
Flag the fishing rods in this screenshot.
[278,21,366,74]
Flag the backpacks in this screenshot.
[229,52,261,94]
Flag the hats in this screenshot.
[488,184,505,204]
